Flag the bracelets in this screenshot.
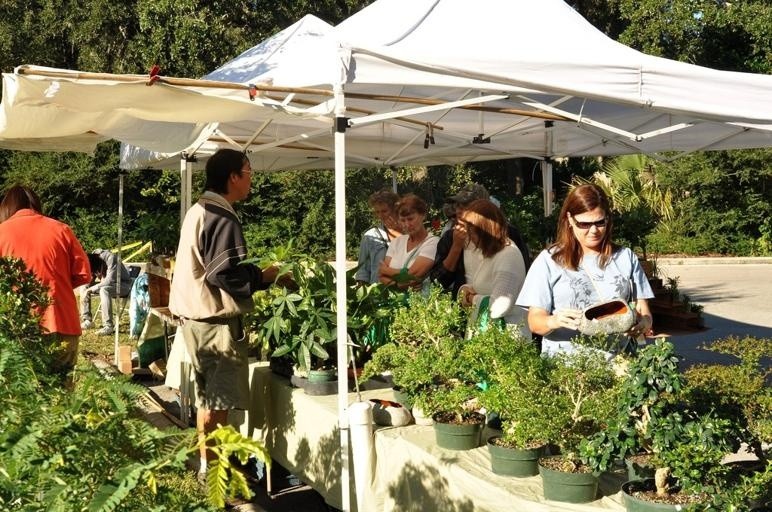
[466,291,470,304]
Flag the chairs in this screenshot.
[92,262,147,329]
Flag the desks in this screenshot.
[141,307,626,512]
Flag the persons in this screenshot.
[456,198,534,343]
[0,182,95,395]
[438,195,459,237]
[79,248,134,338]
[514,182,657,384]
[164,147,294,507]
[377,193,441,300]
[353,188,406,288]
[429,182,529,336]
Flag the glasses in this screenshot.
[241,169,254,177]
[571,215,607,230]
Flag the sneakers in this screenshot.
[79,319,96,329]
[94,326,113,336]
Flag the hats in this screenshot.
[446,183,490,204]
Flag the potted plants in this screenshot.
[354,283,488,450]
[462,326,573,478]
[503,329,627,503]
[620,333,744,511]
[238,240,417,395]
